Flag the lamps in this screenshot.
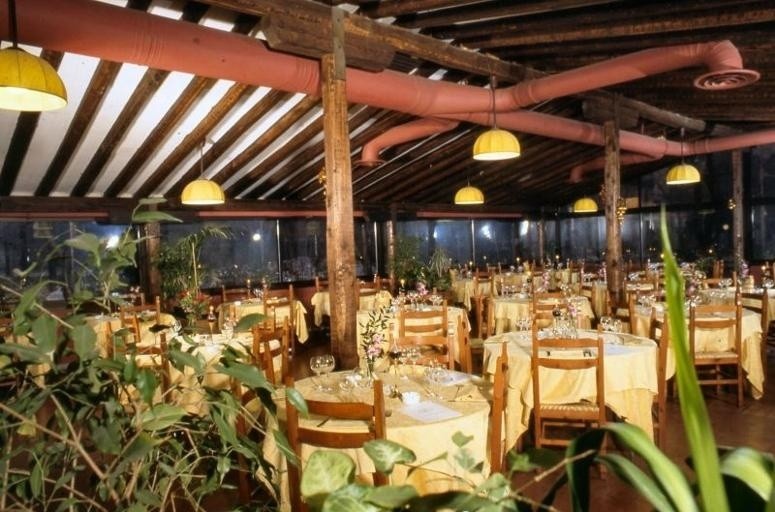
[573,194,598,213]
[181,135,225,205]
[666,128,700,184]
[454,176,485,204]
[473,74,521,162]
[0,0,68,112]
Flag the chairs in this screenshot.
[0,257,775,512]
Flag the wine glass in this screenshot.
[513,309,623,350]
[388,287,443,315]
[583,272,592,285]
[253,288,265,304]
[309,343,446,403]
[765,279,775,296]
[636,291,658,316]
[501,280,571,300]
[169,320,182,338]
[718,278,733,294]
